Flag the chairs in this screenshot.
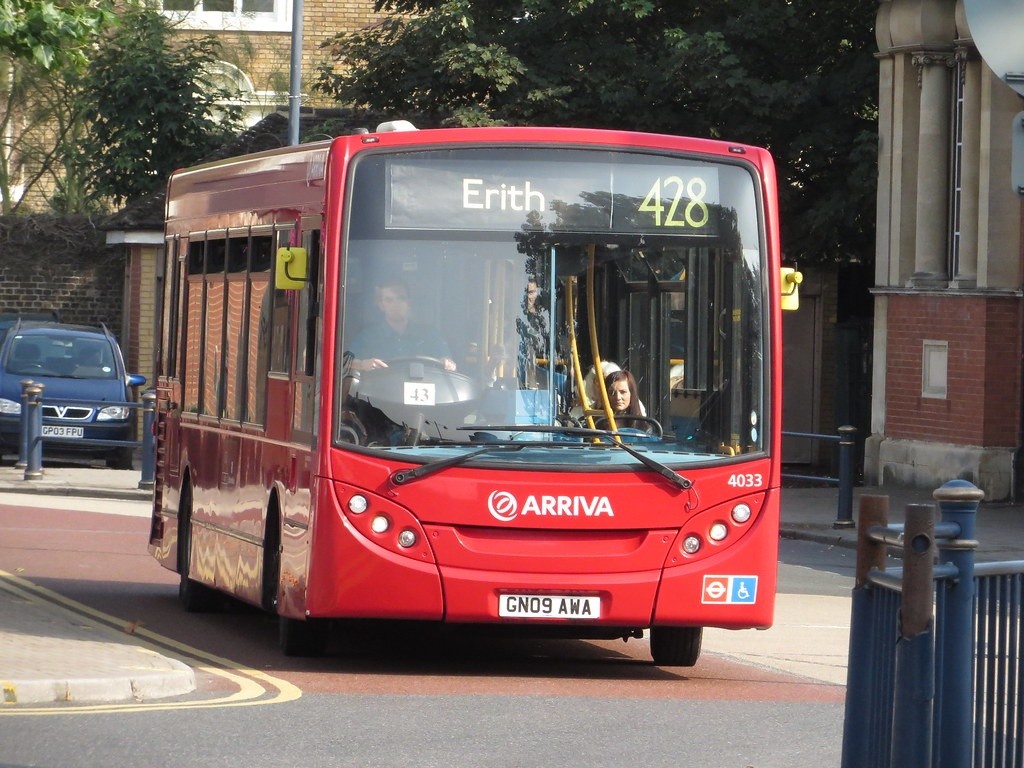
[8,343,46,372]
[61,348,103,376]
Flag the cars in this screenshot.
[0,315,145,471]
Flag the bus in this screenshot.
[147,121,803,667]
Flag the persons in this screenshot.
[513,276,551,385]
[634,420,638,424]
[341,278,456,371]
[63,345,105,377]
[574,370,654,443]
